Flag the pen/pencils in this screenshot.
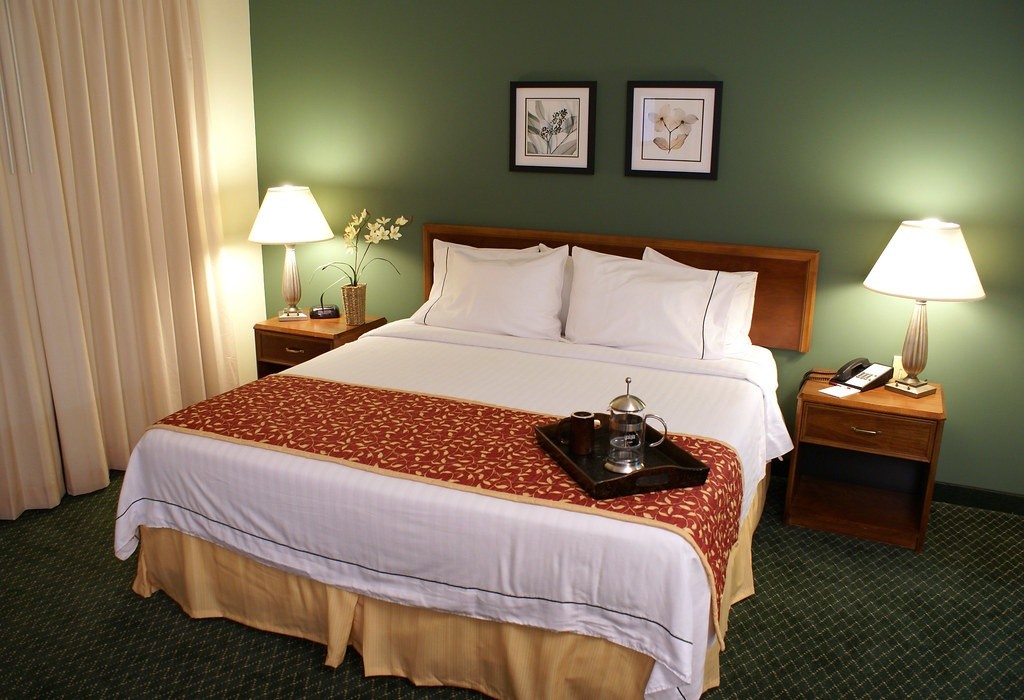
[830,382,861,393]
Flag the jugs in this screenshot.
[604,377,667,474]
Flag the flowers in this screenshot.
[307,209,413,312]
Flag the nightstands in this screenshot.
[784,368,947,553]
[252,308,387,379]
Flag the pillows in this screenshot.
[564,246,761,363]
[409,238,577,345]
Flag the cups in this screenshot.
[556,410,595,456]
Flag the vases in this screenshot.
[342,282,367,326]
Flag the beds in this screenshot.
[115,223,820,700]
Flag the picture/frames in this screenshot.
[623,80,723,181]
[509,81,598,175]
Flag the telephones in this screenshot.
[829,357,894,391]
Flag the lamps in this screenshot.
[862,218,987,399]
[247,185,335,322]
